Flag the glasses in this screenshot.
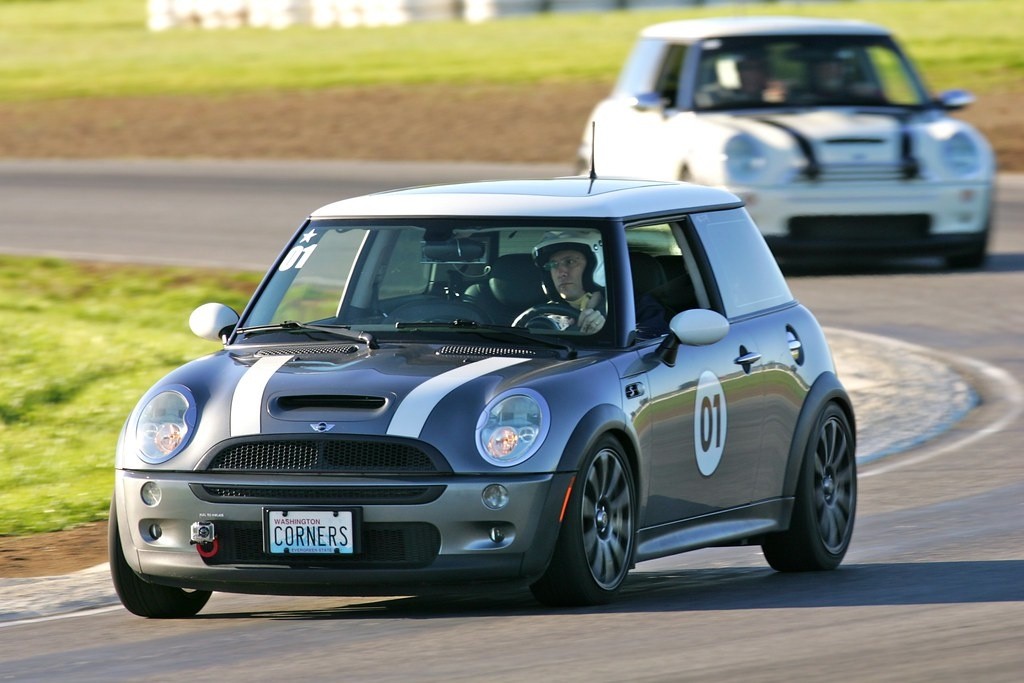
[541,255,584,271]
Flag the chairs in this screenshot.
[464,253,538,324]
[607,253,670,316]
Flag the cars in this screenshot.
[107,175,858,618]
[576,19,995,272]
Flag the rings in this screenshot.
[595,320,600,325]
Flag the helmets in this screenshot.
[532,228,605,291]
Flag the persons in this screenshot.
[806,57,875,98]
[530,228,669,336]
[702,51,788,107]
